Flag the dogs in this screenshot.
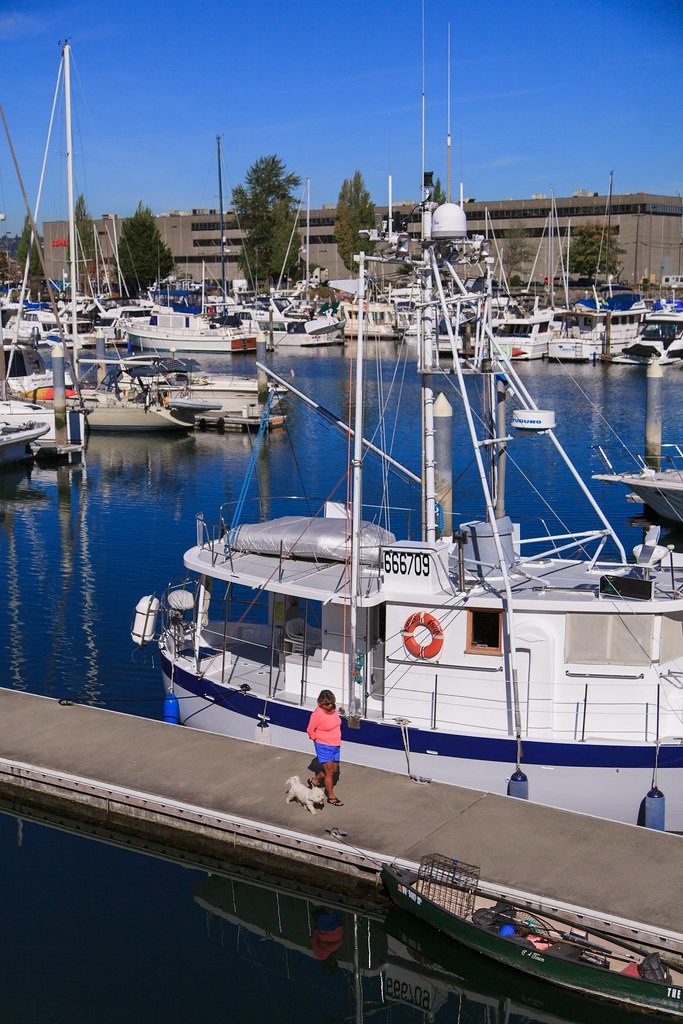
[284,775,325,816]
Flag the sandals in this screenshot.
[328,797,343,807]
[308,778,318,789]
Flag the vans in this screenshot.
[662,275,682,287]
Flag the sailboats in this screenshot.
[0,0,682,833]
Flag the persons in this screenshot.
[307,690,344,806]
[309,905,344,977]
[162,391,172,408]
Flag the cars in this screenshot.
[543,277,552,285]
[554,276,574,287]
[525,281,543,287]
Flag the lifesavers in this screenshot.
[403,611,445,660]
[208,306,215,315]
